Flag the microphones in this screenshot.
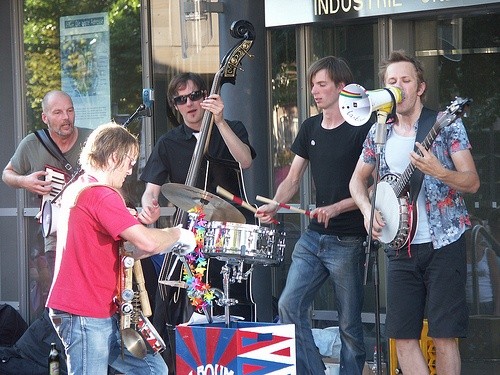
[124,104,146,128]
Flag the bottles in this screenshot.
[48,342,60,375]
[373,341,384,375]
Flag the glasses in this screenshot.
[172,90,204,105]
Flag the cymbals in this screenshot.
[160,183,246,224]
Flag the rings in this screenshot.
[153,203,160,208]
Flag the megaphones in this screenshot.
[338,84,402,126]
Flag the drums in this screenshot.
[200,221,286,266]
[135,309,166,354]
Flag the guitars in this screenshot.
[367,96,474,251]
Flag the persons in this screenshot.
[465,216,500,316]
[348,50,480,375]
[45,122,169,375]
[255,57,377,375]
[2,90,95,284]
[138,73,257,375]
